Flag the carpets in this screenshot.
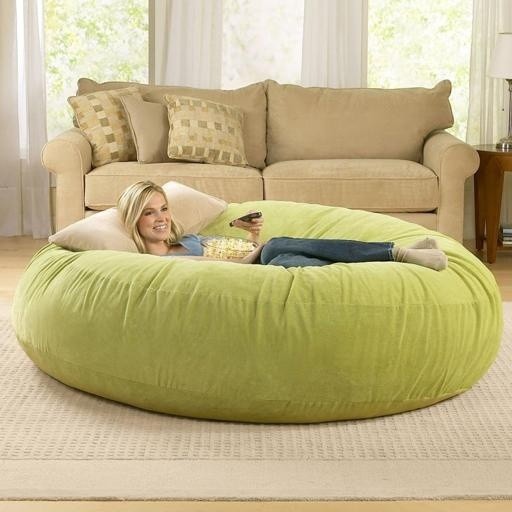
[0,302,512,500]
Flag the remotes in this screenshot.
[228,211,262,227]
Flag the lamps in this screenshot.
[488,34,512,149]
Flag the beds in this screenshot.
[11,200,503,425]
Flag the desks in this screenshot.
[474,145,512,264]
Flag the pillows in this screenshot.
[117,94,183,166]
[67,85,144,166]
[162,93,249,166]
[265,79,453,164]
[72,78,269,167]
[47,180,229,252]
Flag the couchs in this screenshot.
[39,79,481,245]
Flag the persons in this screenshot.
[114,177,449,276]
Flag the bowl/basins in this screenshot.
[200,236,258,259]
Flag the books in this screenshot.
[499,233,512,241]
[500,223,512,234]
[498,239,512,245]
